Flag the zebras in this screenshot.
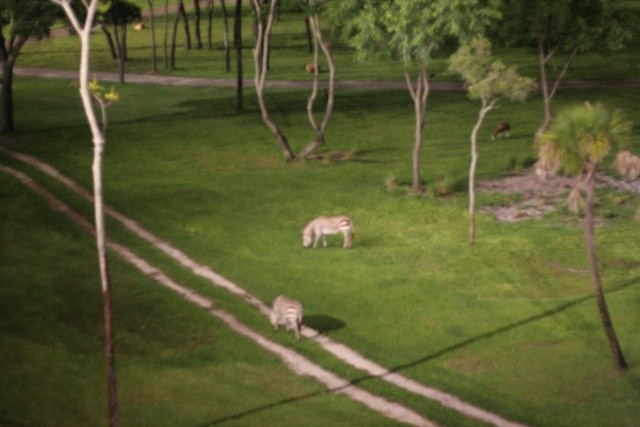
[302,215,353,250]
[270,295,303,341]
